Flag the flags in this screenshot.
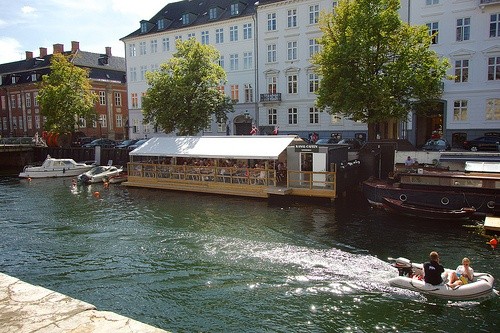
[274,128,278,135]
[312,133,317,141]
[250,126,256,135]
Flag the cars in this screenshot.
[74,136,148,151]
[421,137,451,152]
[311,137,364,148]
[463,135,500,152]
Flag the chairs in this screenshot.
[134,164,278,185]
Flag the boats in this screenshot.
[18,154,127,185]
[388,257,495,300]
[364,161,500,224]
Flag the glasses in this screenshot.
[463,264,469,266]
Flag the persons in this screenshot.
[446,258,474,289]
[423,251,448,285]
[142,156,287,182]
[405,156,413,166]
